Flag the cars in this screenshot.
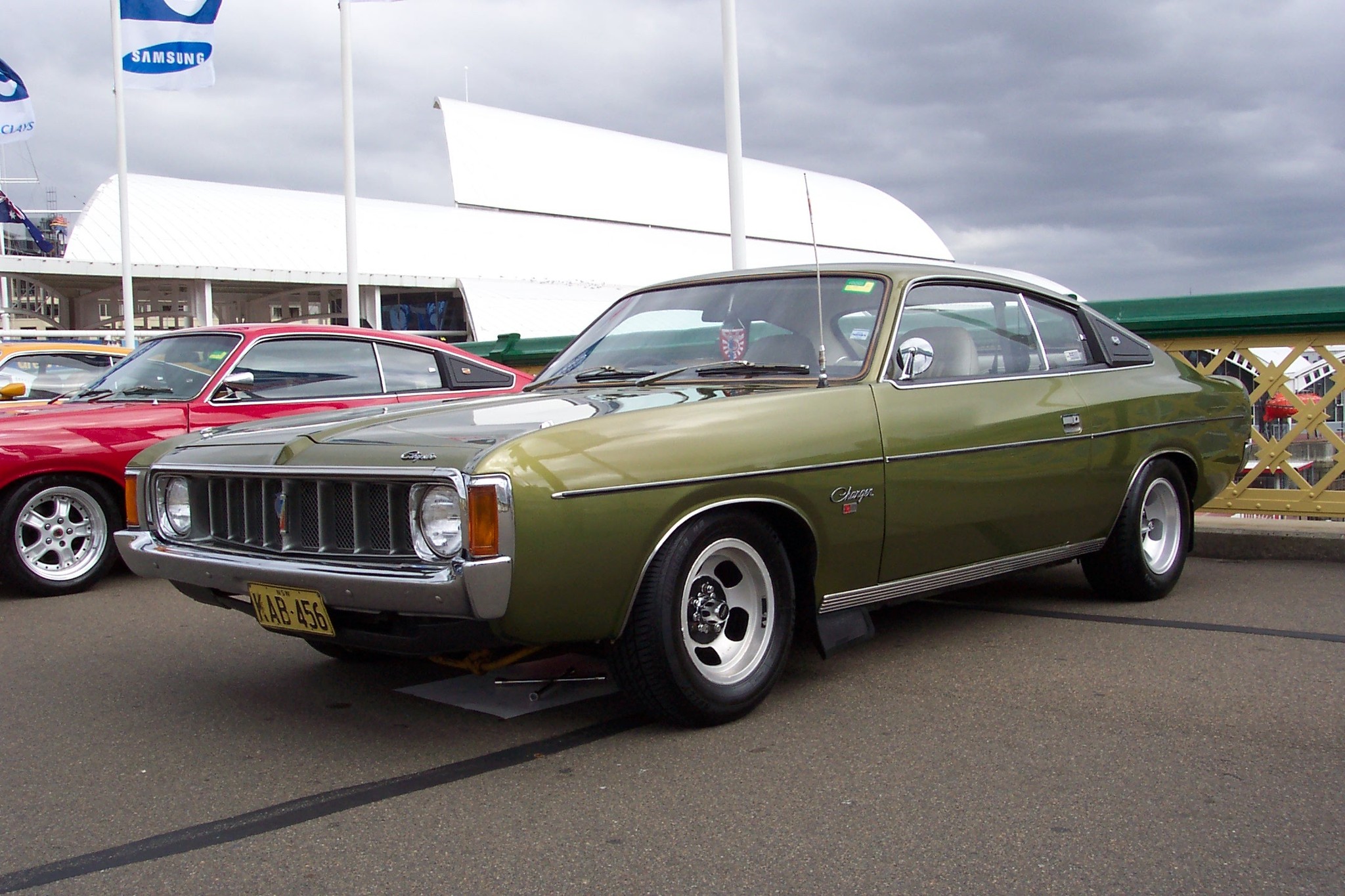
[0,323,545,597]
[0,339,211,410]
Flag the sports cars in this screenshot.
[112,263,1256,722]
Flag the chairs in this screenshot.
[895,326,979,379]
[747,333,818,372]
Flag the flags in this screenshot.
[0,59,40,144]
[0,190,54,253]
[49,217,67,236]
[113,0,222,94]
